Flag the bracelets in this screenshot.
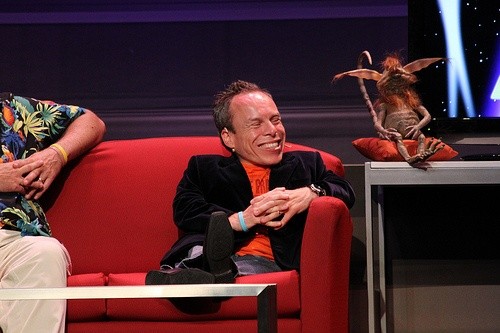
[238,211,249,232]
[48,147,63,168]
[49,143,68,166]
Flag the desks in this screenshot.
[0,283,277,333]
[364,159,500,333]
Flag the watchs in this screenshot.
[306,183,326,198]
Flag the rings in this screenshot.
[39,177,44,184]
[2,154,9,163]
[276,210,281,216]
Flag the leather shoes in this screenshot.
[202,211,236,284]
[144,267,215,285]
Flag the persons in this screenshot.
[143,77,358,316]
[0,88,107,333]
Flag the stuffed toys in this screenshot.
[330,48,451,172]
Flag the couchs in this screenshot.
[39,133,352,333]
